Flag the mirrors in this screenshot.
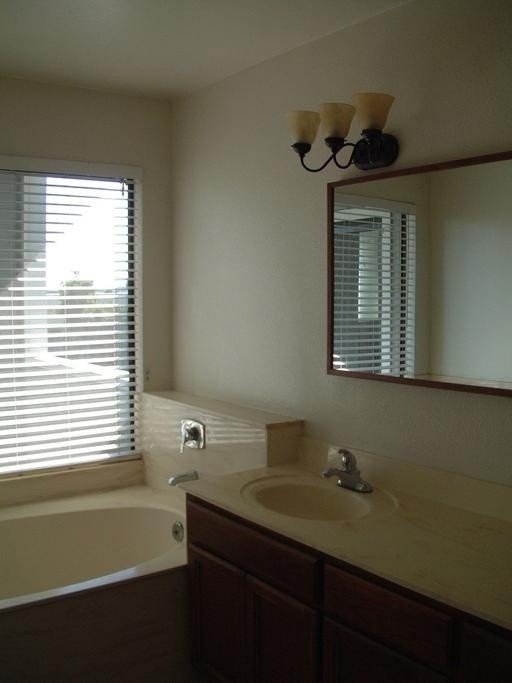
[323,144,512,401]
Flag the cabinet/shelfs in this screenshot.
[176,492,512,680]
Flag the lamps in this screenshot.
[283,87,400,170]
[356,208,379,321]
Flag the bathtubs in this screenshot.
[1,485,189,611]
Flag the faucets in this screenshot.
[320,448,374,493]
[166,469,199,487]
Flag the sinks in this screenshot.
[239,471,399,525]
